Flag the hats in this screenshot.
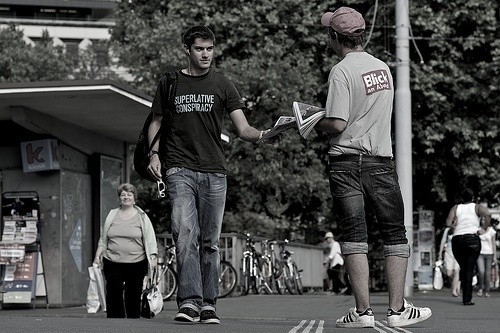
[324,232,334,240]
[320,6,365,36]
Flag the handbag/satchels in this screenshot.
[141,286,163,319]
[433,262,442,290]
[86,262,107,313]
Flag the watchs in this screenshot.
[147,151,159,158]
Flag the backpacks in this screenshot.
[134,72,179,182]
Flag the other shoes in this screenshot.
[477,289,483,297]
[463,300,475,305]
[453,291,460,296]
[485,292,491,297]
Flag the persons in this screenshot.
[446,189,490,305]
[314,6,432,328]
[93,184,157,319]
[323,231,348,295]
[146,26,286,323]
[438,217,500,296]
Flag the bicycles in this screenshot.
[152,244,238,302]
[239,230,304,295]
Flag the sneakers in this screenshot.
[336,305,374,328]
[386,297,432,328]
[173,307,200,323]
[199,310,220,323]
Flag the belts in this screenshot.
[329,156,391,163]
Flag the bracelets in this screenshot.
[260,130,265,143]
